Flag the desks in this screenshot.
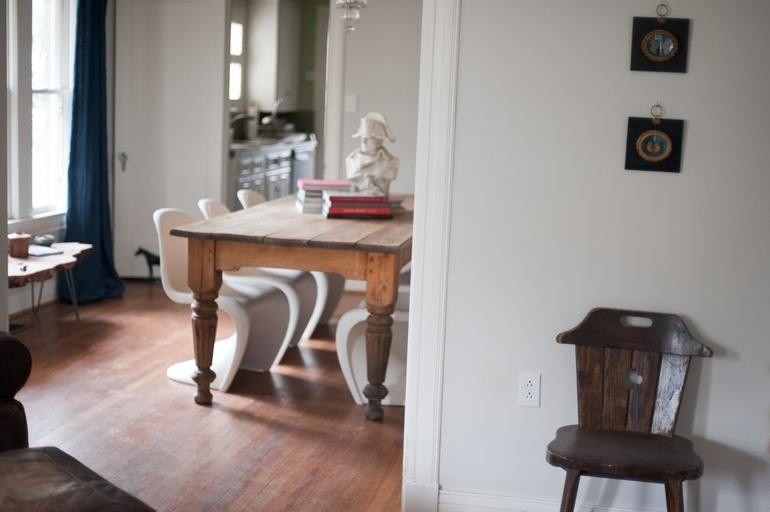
[4,241,95,336]
[170,194,414,421]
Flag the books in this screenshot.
[322,191,394,218]
[296,178,352,214]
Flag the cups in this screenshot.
[7,231,31,257]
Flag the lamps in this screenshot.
[333,1,369,34]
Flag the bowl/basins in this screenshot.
[32,234,55,246]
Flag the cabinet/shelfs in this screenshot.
[266,146,291,200]
[246,1,325,113]
[229,140,265,206]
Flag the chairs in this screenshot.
[197,198,317,366]
[546,307,713,510]
[335,292,422,407]
[153,208,290,392]
[237,189,344,340]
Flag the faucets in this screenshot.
[271,96,285,120]
[229,114,256,124]
